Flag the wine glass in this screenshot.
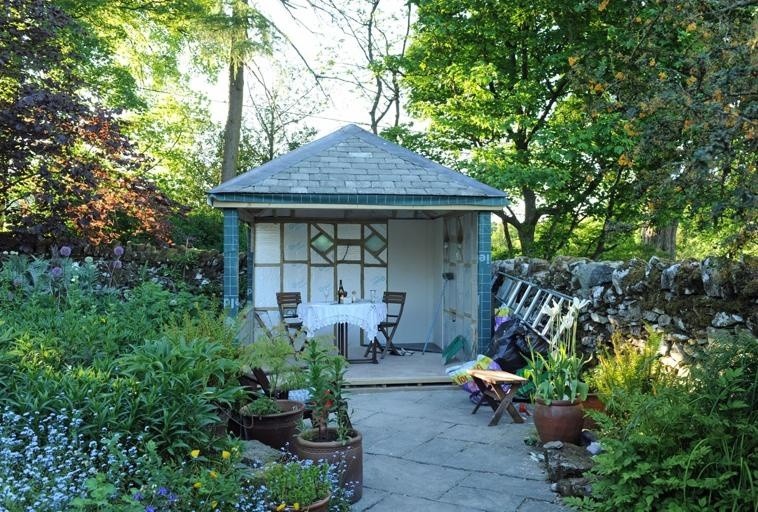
[322,289,330,303]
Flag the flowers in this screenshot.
[510,296,594,406]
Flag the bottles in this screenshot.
[339,279,344,303]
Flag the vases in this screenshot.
[534,398,582,447]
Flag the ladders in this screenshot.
[491,270,574,350]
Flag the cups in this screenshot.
[370,289,377,302]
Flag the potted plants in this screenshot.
[261,462,332,512]
[296,338,362,505]
[234,319,306,456]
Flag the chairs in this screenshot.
[364,291,406,359]
[276,292,310,352]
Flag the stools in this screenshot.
[467,368,529,426]
